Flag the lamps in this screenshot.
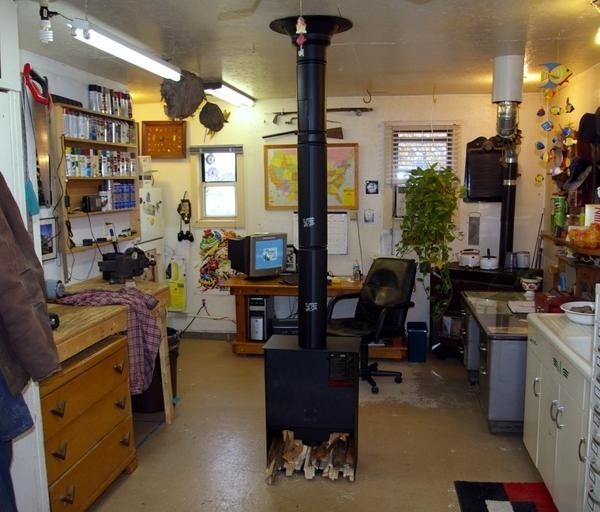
[69,0,183,85]
[35,0,56,47]
[204,81,255,108]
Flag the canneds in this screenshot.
[352,265,359,279]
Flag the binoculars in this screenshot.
[178,230,194,242]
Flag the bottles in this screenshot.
[505,251,518,268]
[118,226,138,239]
[351,257,360,280]
[104,220,115,242]
[61,84,135,212]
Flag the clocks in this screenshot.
[142,119,188,161]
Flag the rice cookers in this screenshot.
[455,247,481,269]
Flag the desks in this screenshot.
[459,288,566,436]
[41,273,177,426]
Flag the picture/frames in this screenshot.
[37,216,57,263]
[261,141,360,213]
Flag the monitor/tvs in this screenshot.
[228,233,286,281]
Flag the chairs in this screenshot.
[319,256,419,395]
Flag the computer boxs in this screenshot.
[248,296,274,341]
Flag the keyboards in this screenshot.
[283,277,299,285]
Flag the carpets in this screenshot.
[451,479,560,512]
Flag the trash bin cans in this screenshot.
[406,321,428,362]
[130,326,180,414]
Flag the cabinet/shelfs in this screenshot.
[578,429,590,511]
[221,272,413,363]
[422,257,545,362]
[39,334,139,512]
[534,367,583,512]
[522,347,542,469]
[48,102,143,254]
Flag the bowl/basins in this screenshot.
[558,301,597,327]
[521,275,543,291]
[96,237,107,243]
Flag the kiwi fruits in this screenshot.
[570,305,592,315]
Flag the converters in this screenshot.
[64,195,70,207]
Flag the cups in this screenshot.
[83,239,93,247]
[355,270,364,284]
[515,251,532,269]
[330,276,342,287]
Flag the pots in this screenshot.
[478,247,497,271]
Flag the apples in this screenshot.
[568,223,600,248]
